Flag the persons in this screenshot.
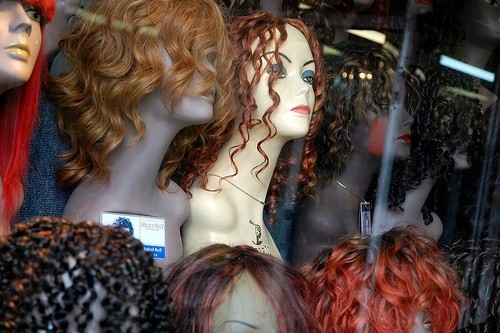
[0,0,500,333]
